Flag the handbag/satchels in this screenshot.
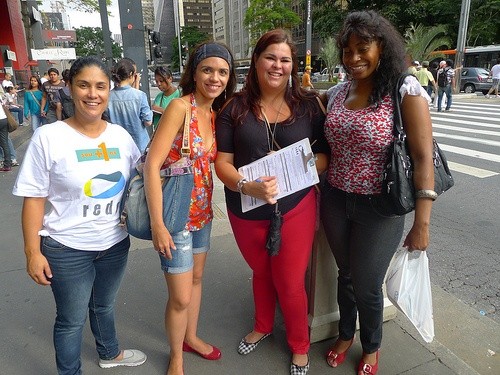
[119,157,194,240]
[381,134,454,216]
[385,247,435,343]
[2,105,19,132]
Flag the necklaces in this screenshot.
[258,98,285,151]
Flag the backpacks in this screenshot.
[438,67,450,87]
[58,88,75,118]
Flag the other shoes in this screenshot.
[290,352,311,375]
[0,166,12,173]
[182,341,221,361]
[237,332,273,355]
[10,159,19,166]
[98,349,148,368]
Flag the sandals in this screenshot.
[326,336,354,367]
[357,349,380,375]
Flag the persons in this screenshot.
[485,58,500,99]
[106,58,153,155]
[438,59,456,112]
[143,41,238,375]
[24,67,75,133]
[318,10,435,375]
[301,65,314,91]
[430,62,439,104]
[0,84,20,172]
[213,28,330,375]
[1,73,30,127]
[151,66,184,132]
[434,61,446,108]
[415,61,437,92]
[12,56,147,375]
[406,61,420,75]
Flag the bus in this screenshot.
[234,67,251,84]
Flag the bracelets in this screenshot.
[414,190,438,201]
[237,177,249,196]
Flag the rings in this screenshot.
[160,251,165,255]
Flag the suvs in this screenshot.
[311,66,347,83]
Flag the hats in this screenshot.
[414,61,419,65]
[421,61,429,65]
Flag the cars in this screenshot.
[460,67,494,93]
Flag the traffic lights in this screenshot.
[147,28,163,61]
